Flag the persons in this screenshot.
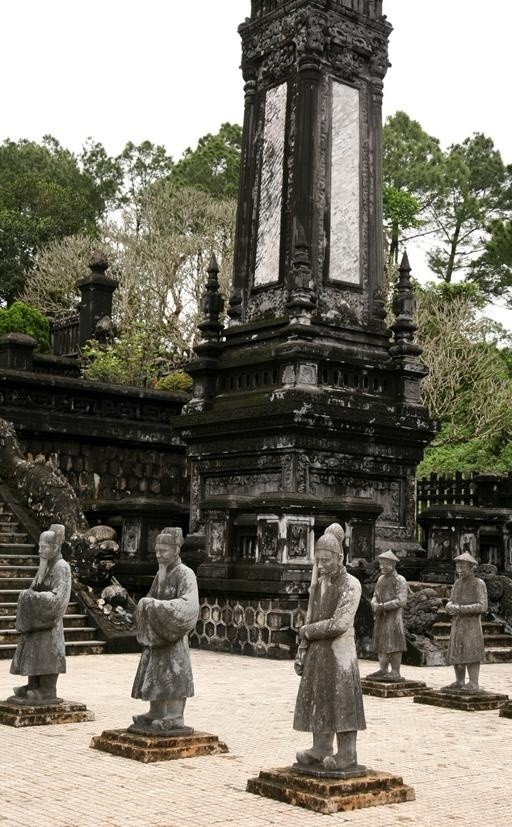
[291,522,367,768]
[443,549,491,691]
[368,547,409,680]
[7,523,72,700]
[131,526,200,730]
